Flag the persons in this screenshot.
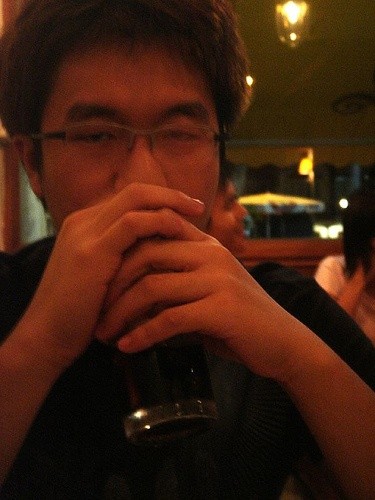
[205,156,249,261]
[0,0,375,499]
[314,181,372,344]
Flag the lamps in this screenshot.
[273,0,312,49]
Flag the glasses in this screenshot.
[25,115,233,170]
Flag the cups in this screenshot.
[106,234,220,447]
